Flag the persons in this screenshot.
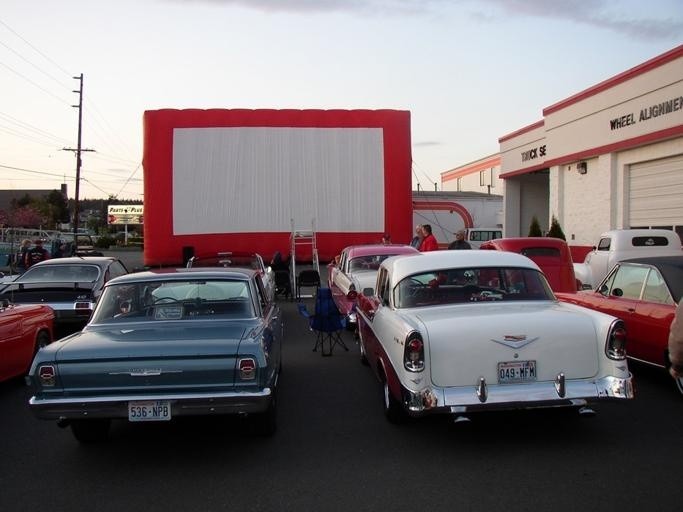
[417,224,436,252]
[16,238,31,275]
[407,224,424,250]
[376,233,395,262]
[50,232,63,258]
[667,295,683,379]
[445,231,472,283]
[24,240,47,271]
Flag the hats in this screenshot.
[453,230,465,237]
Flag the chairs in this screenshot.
[275,270,349,355]
